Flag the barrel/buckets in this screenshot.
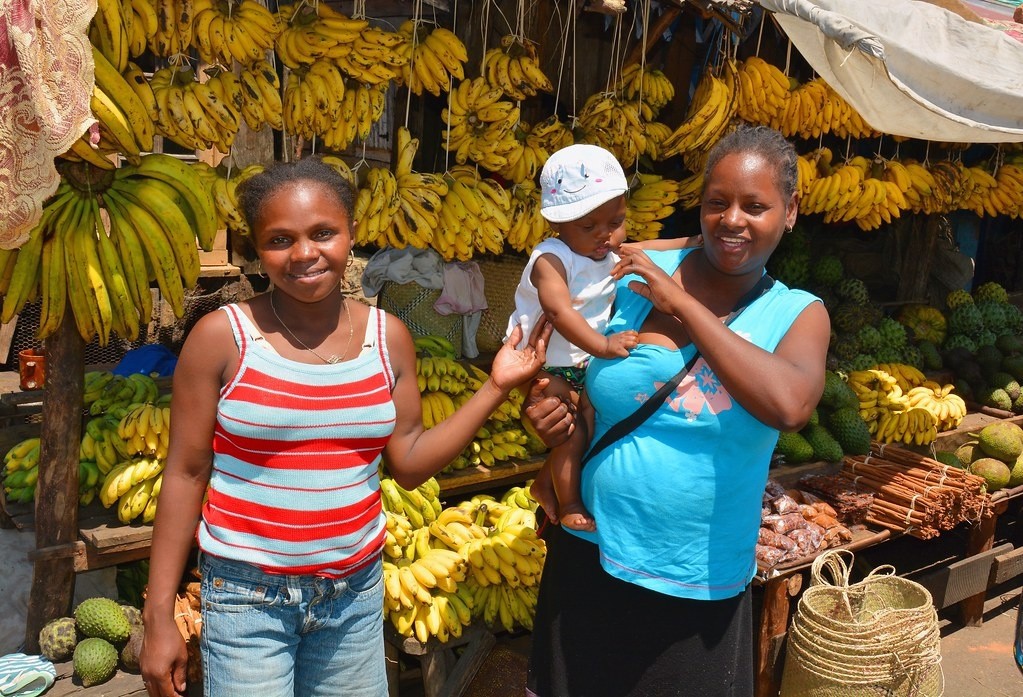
[18,347,45,391]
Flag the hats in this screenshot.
[540,144,629,222]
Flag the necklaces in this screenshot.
[270,291,353,364]
[671,315,684,327]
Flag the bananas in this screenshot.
[1,369,171,526]
[378,461,545,645]
[845,363,966,446]
[4,0,1023,345]
[412,333,547,475]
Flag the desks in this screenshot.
[27,397,1023,697]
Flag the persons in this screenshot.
[501,144,704,532]
[519,127,830,696]
[138,157,555,697]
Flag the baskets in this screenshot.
[780,548,945,697]
